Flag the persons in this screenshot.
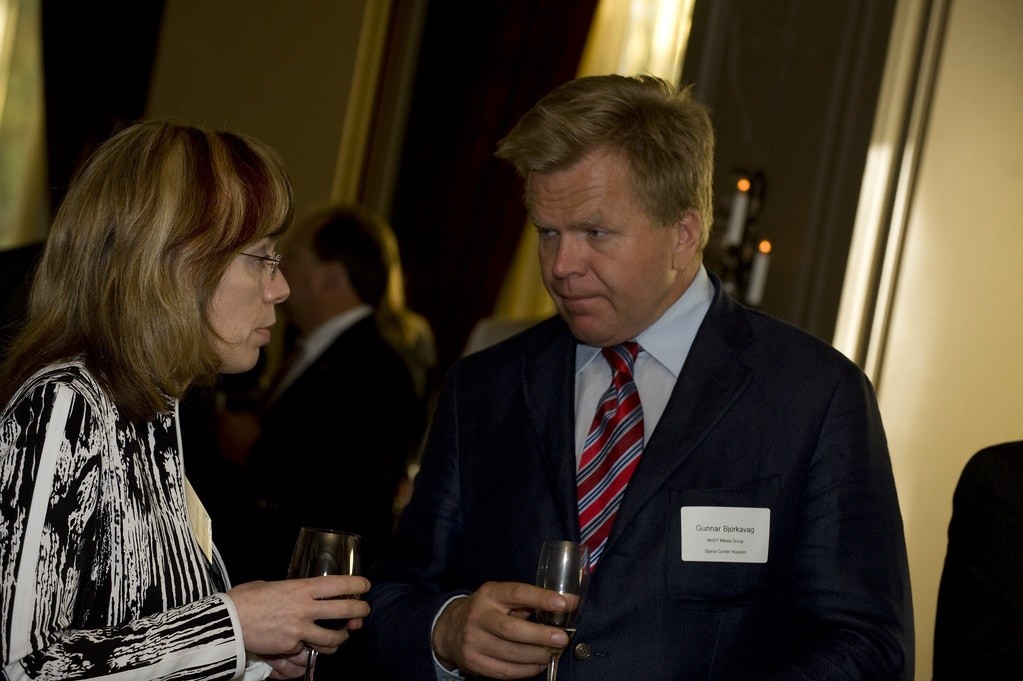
[0,121,371,681]
[930,440,1023,681]
[212,206,425,556]
[363,76,914,681]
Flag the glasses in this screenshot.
[237,252,282,281]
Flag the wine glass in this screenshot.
[285,528,361,681]
[536,540,591,681]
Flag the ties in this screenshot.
[266,345,302,405]
[576,342,645,577]
